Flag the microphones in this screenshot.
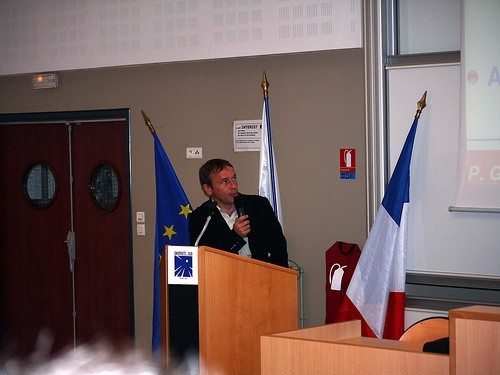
[234,196,247,224]
[194,203,216,247]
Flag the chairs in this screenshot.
[398,317,449,343]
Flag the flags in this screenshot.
[259,99,284,233]
[152,133,193,369]
[334,118,418,340]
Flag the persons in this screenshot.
[188,159,289,268]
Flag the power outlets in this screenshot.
[186,147,203,159]
